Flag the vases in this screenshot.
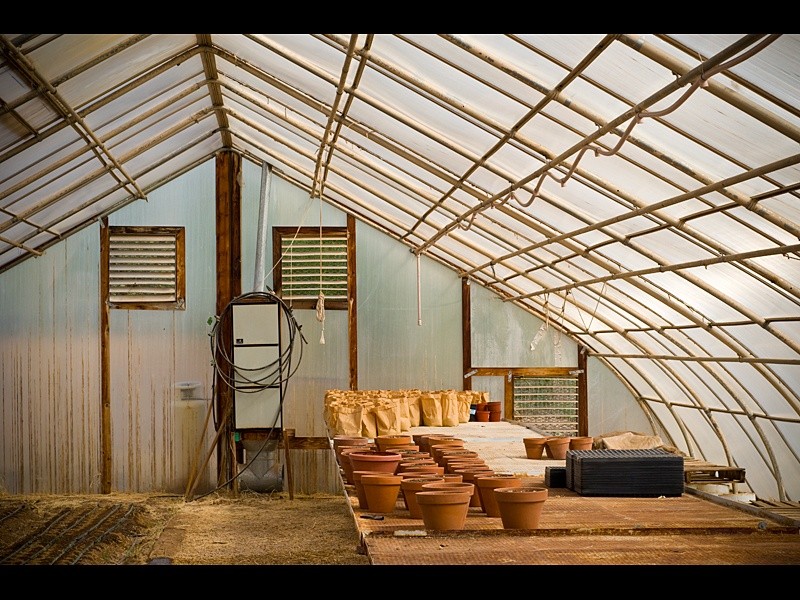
[333,434,594,530]
[476,401,503,422]
